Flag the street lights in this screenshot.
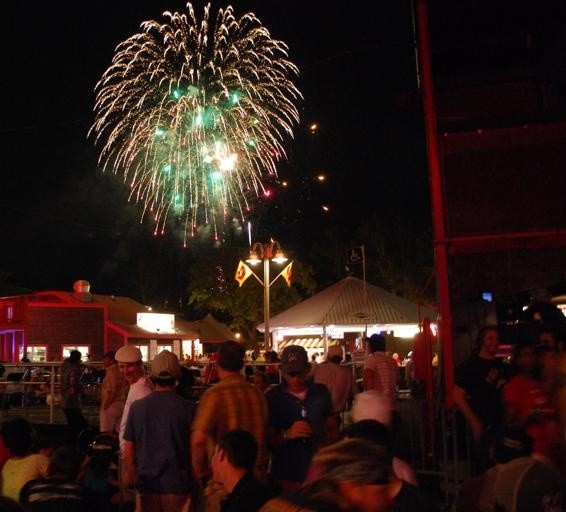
[246,240,288,354]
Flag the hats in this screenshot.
[327,346,343,360]
[152,350,179,380]
[115,347,142,363]
[351,391,392,426]
[281,346,309,375]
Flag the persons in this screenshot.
[1,318,433,511]
[453,302,564,510]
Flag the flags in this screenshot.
[282,262,294,287]
[235,261,253,286]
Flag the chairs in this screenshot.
[0,433,119,511]
[0,359,213,411]
